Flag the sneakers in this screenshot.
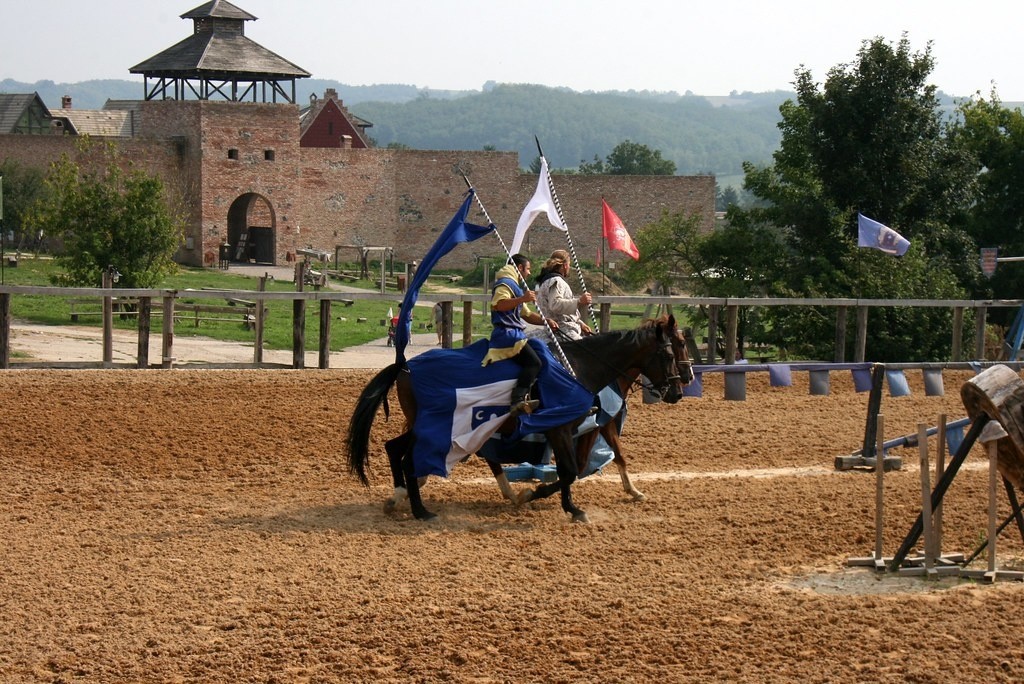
[510,396,540,417]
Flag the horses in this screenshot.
[342,320,684,525]
[401,314,696,504]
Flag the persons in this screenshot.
[398,302,413,345]
[482,253,560,415]
[430,301,443,346]
[536,248,593,341]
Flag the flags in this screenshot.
[602,199,639,263]
[858,213,912,257]
[398,188,497,339]
[980,247,998,280]
[507,155,567,266]
